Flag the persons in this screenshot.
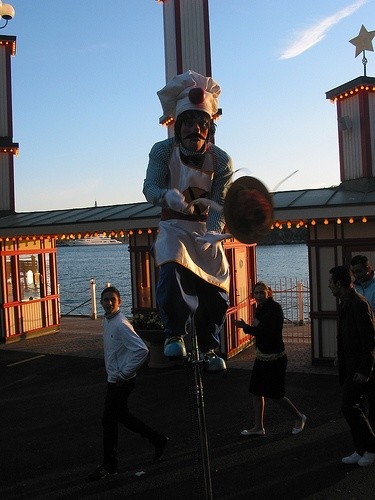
[142,68,231,370]
[86,287,169,480]
[233,281,307,436]
[329,266,375,466]
[350,255,375,315]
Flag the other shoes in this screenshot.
[292,414,306,434]
[241,428,266,434]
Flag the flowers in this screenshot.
[126,311,165,332]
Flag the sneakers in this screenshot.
[153,434,170,461]
[88,465,118,479]
[342,451,361,463]
[358,452,375,466]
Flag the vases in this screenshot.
[138,330,162,343]
